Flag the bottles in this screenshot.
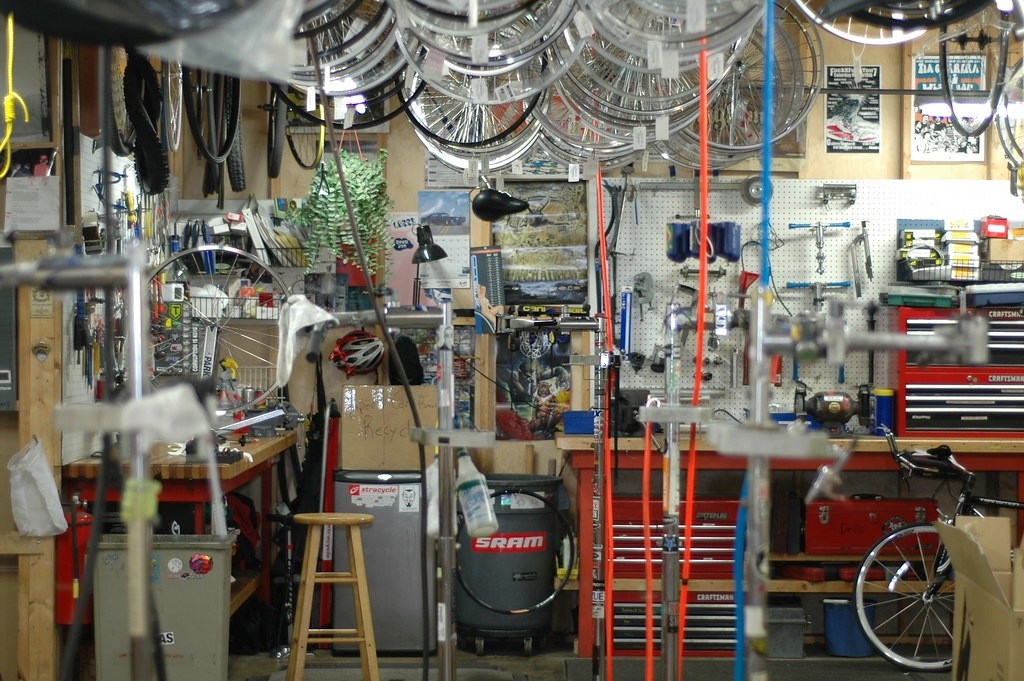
[456,447,498,538]
[166,235,189,308]
[97,373,104,400]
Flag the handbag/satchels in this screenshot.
[177,491,259,570]
[7,438,68,537]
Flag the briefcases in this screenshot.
[772,480,788,553]
[804,492,939,555]
[787,490,801,554]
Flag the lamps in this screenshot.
[410,222,449,311]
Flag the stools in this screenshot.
[285,513,380,681]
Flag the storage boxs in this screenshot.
[933,516,1024,681]
[341,384,437,472]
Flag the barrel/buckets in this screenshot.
[456,473,562,636]
[819,598,875,657]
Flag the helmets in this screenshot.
[329,330,385,380]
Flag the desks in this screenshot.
[60,429,298,621]
[553,429,1024,681]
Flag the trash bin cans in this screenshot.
[331,469,436,654]
[91,531,239,680]
[455,474,565,658]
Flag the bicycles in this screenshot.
[851,422,1024,673]
